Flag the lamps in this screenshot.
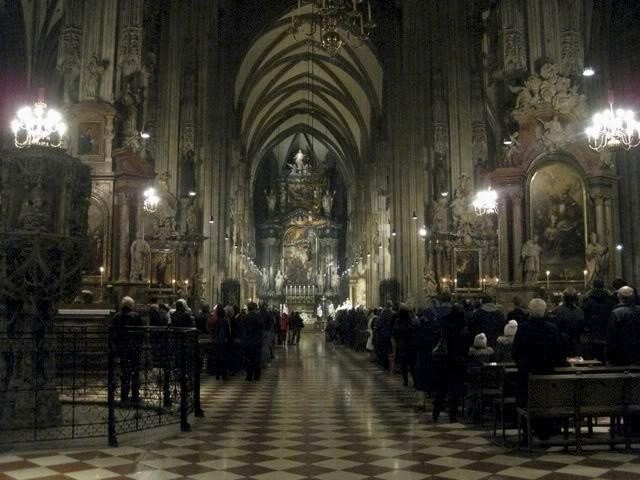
[143,188,161,213]
[471,186,498,216]
[10,102,68,149]
[289,0,377,59]
[581,102,640,152]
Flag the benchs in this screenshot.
[472,360,639,456]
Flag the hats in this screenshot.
[504,320,518,336]
[614,286,633,296]
[474,333,487,347]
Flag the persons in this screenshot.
[107,293,306,405]
[582,231,609,289]
[432,286,640,440]
[326,299,413,371]
[520,232,546,282]
[126,229,157,281]
[83,54,106,97]
